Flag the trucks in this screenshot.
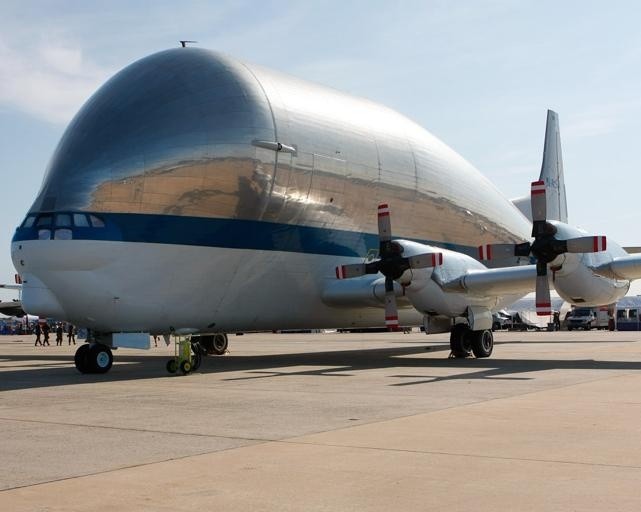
[566,307,609,330]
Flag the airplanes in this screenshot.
[0,40,641,374]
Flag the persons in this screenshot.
[55,325,62,346]
[67,324,75,346]
[33,323,42,346]
[40,323,50,346]
